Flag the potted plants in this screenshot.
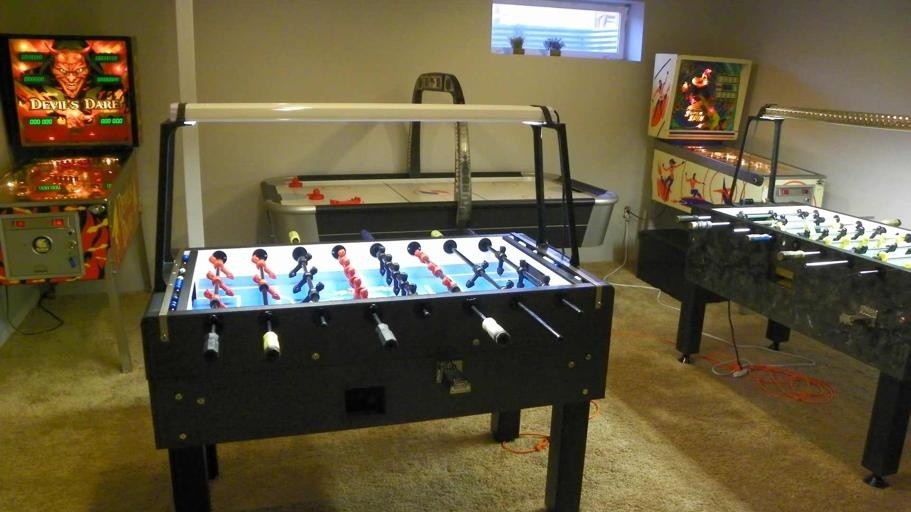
[510,36,525,54]
[543,36,565,56]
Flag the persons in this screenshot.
[660,158,686,200]
[651,70,672,129]
[684,171,706,202]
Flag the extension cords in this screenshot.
[734,367,750,377]
